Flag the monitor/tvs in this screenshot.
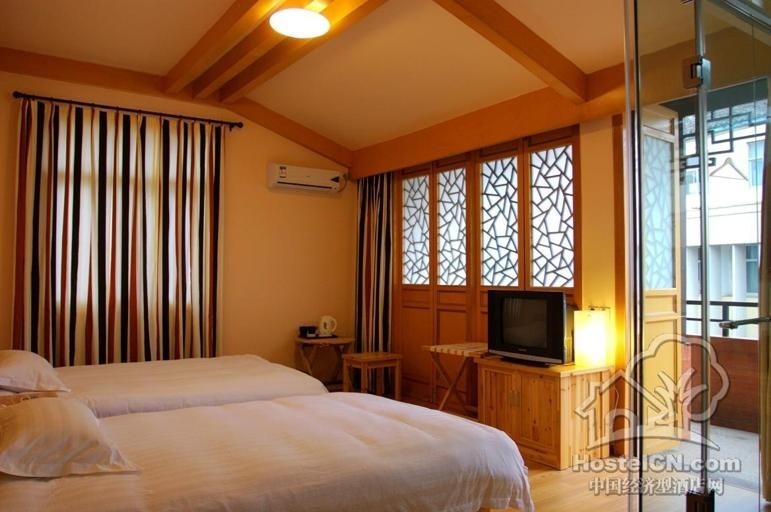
[483,287,573,369]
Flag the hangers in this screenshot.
[709,156,749,182]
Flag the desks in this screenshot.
[293,336,355,392]
[421,341,489,417]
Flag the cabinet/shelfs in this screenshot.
[473,357,610,471]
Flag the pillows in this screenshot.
[0,349,66,391]
[1,392,138,478]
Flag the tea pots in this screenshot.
[318,315,337,337]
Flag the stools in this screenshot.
[341,351,403,403]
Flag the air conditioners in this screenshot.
[268,162,346,194]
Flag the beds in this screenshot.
[1,391,536,512]
[0,352,328,417]
